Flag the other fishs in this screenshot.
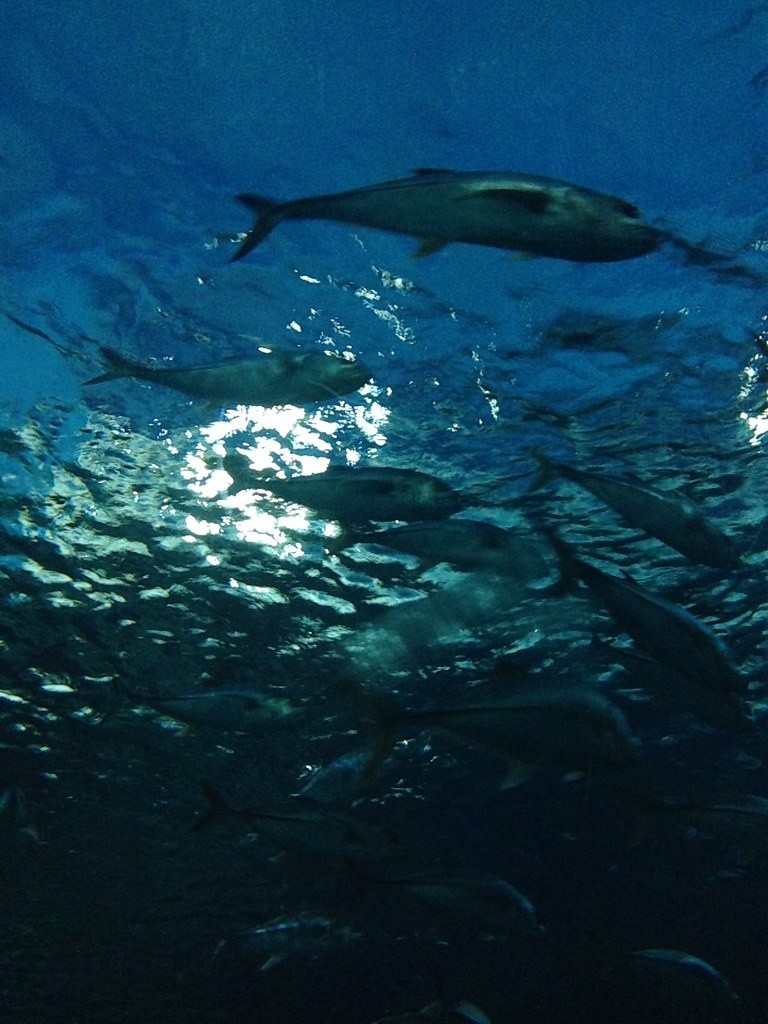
[102,444,755,861]
[81,347,377,408]
[363,958,491,1023]
[332,860,545,948]
[202,902,377,973]
[226,164,677,268]
[627,945,741,1015]
